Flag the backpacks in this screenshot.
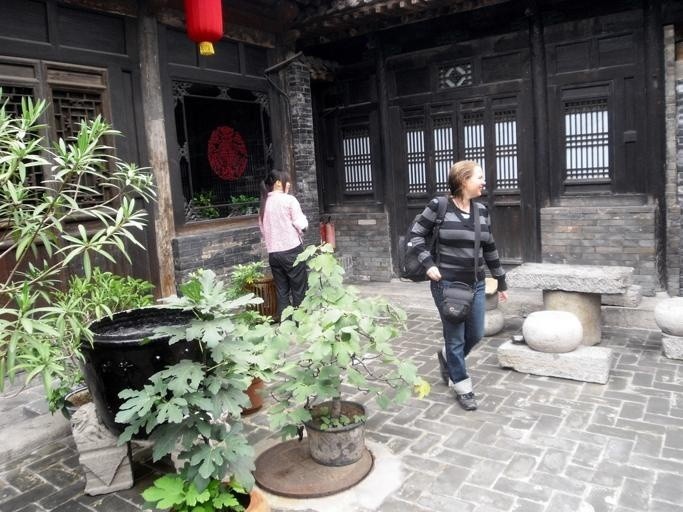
[394,195,447,283]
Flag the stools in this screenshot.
[482,278,503,336]
[654,296,683,362]
[499,307,609,383]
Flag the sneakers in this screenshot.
[455,389,478,411]
[437,348,450,388]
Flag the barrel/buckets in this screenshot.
[78,305,217,441]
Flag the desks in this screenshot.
[505,262,633,346]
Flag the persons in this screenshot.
[410,160,508,410]
[257,170,309,327]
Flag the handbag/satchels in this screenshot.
[438,278,476,325]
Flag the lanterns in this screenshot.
[184,0,224,57]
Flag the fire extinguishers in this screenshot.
[319,215,336,253]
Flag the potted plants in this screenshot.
[266,240,430,467]
[40,267,156,419]
[116,263,266,512]
